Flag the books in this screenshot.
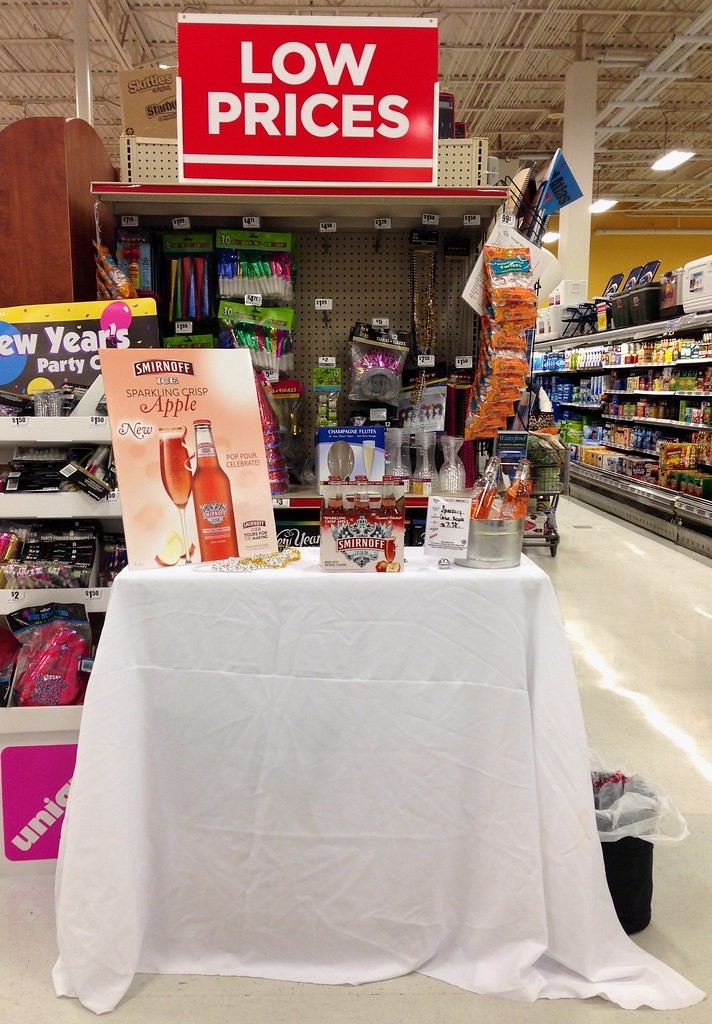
[476,148,583,249]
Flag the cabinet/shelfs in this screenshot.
[533,313,712,561]
[0,416,127,861]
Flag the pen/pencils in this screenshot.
[70,461,113,492]
[0,565,81,588]
[107,545,126,587]
[85,445,108,473]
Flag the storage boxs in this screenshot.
[580,447,657,477]
[668,377,695,391]
[314,427,387,495]
[117,65,177,139]
[611,255,712,329]
[606,403,712,426]
[627,376,640,389]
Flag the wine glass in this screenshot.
[160,426,201,566]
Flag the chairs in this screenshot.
[560,272,625,338]
[595,260,660,328]
[579,265,642,333]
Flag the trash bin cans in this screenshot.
[630,283,662,323]
[592,771,653,936]
[609,291,633,328]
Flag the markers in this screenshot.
[18,628,92,706]
[0,528,28,562]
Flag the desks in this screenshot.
[54,547,712,1011]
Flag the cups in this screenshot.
[363,440,375,478]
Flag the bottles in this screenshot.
[470,456,501,519]
[192,420,240,563]
[501,459,530,518]
[630,332,712,362]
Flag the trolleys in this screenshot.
[521,438,572,558]
